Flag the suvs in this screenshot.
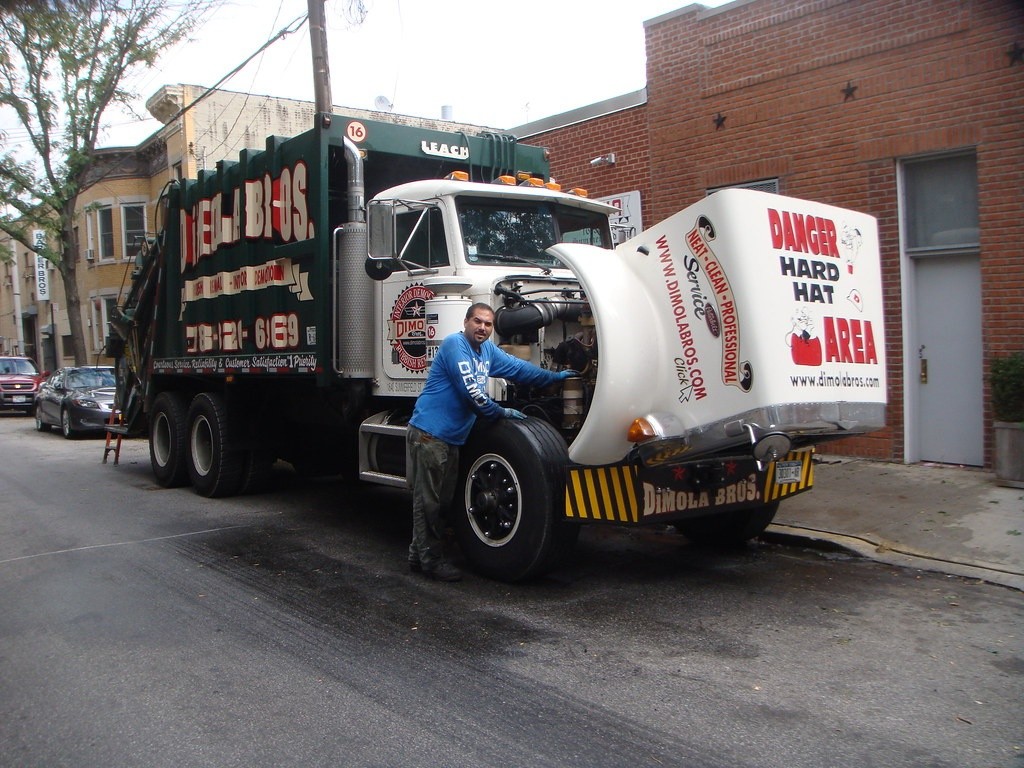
[1,353,50,417]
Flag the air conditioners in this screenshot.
[84,249,94,260]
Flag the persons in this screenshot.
[405,302,579,582]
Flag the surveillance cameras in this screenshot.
[591,157,602,166]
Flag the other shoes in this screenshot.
[420,558,462,581]
[408,553,459,571]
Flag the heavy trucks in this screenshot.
[103,114,888,586]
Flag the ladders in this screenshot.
[103,402,124,466]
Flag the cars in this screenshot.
[34,365,124,440]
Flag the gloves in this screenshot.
[559,369,579,381]
[504,408,527,420]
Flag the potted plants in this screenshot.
[988,350,1024,489]
